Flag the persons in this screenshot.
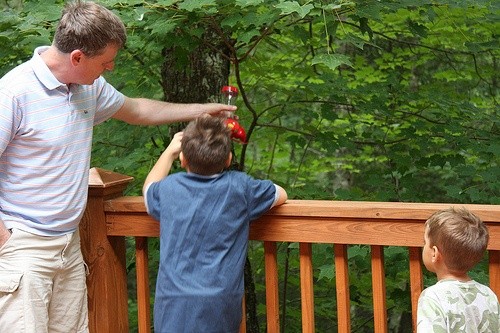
[416,207,500,333]
[142,113,289,333]
[0,2,240,333]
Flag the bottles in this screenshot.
[220,85,247,144]
